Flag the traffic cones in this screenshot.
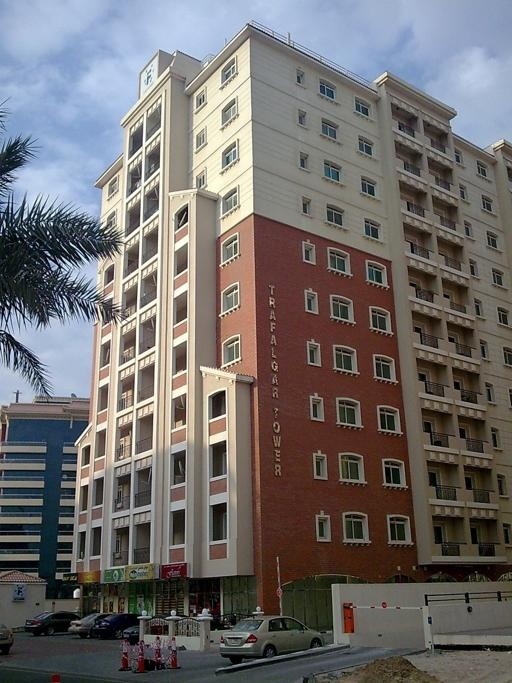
[120,636,181,673]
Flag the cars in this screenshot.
[23,610,187,646]
[219,615,326,664]
[0,622,15,655]
